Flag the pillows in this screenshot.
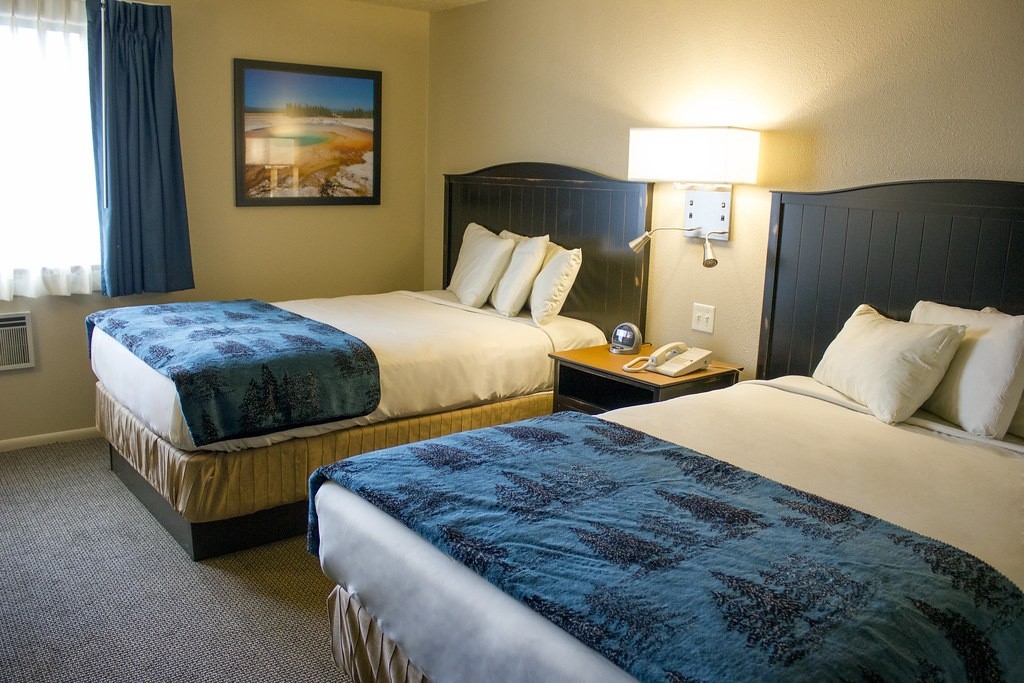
[810,305,968,425]
[484,229,550,315]
[521,241,582,324]
[443,221,516,309]
[910,299,1024,443]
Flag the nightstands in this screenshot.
[547,341,745,421]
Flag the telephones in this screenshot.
[645,341,713,378]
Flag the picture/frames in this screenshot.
[232,58,383,208]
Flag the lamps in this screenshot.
[627,189,733,268]
[625,124,760,183]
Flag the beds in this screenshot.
[306,174,1024,683]
[86,162,653,562]
